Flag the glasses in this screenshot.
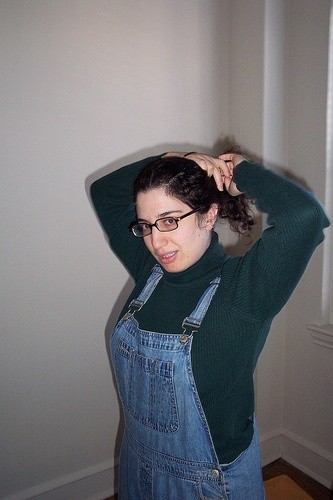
[129,200,210,238]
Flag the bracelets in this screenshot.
[181,151,196,159]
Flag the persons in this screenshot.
[88,150,332,500]
